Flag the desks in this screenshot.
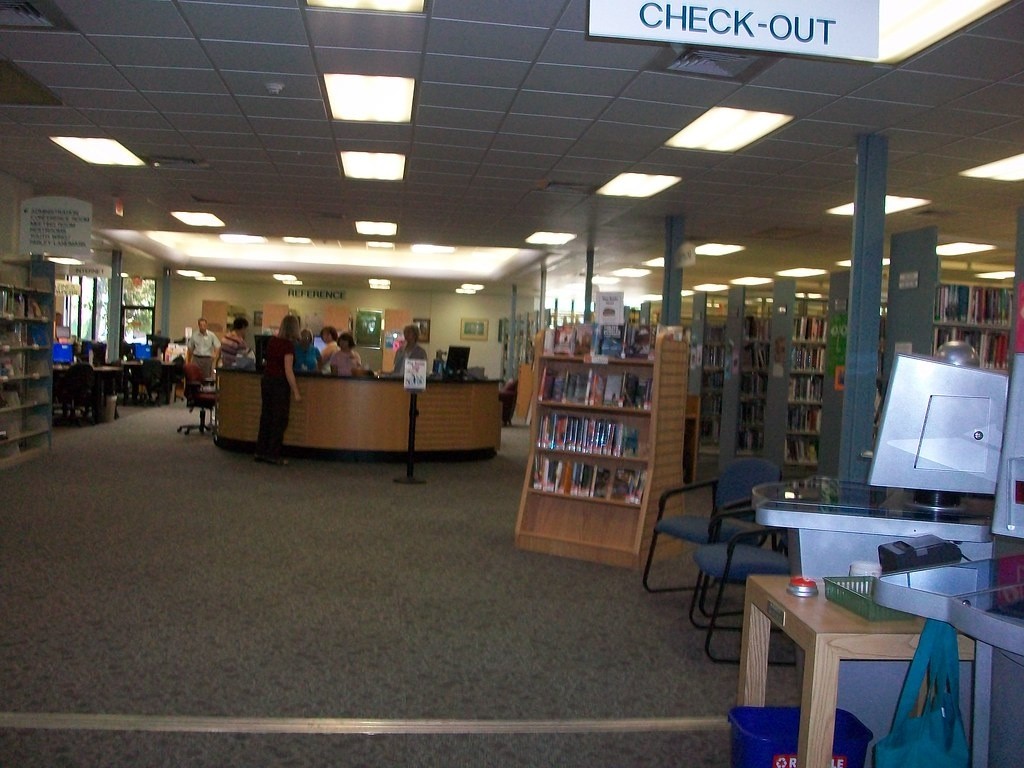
[735,575,976,768]
[53,366,123,419]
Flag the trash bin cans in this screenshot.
[728,706,874,768]
[99,395,117,422]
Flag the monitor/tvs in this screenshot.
[442,347,471,381]
[134,345,152,361]
[52,343,74,365]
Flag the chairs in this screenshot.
[52,361,95,428]
[640,455,798,667]
[125,360,219,435]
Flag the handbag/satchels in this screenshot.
[873,618,970,768]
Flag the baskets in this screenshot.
[823,576,919,622]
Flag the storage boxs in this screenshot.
[729,704,874,767]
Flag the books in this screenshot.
[504,284,1013,504]
[0,290,49,375]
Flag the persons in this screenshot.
[189,318,220,378]
[297,330,322,372]
[319,326,338,373]
[393,325,427,375]
[255,315,301,465]
[330,333,361,376]
[132,348,160,400]
[221,318,250,366]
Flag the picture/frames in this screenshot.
[460,317,489,341]
[413,319,430,342]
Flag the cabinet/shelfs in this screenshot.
[514,280,1016,569]
[0,259,53,469]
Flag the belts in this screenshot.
[193,354,211,358]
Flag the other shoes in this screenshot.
[254,454,290,466]
